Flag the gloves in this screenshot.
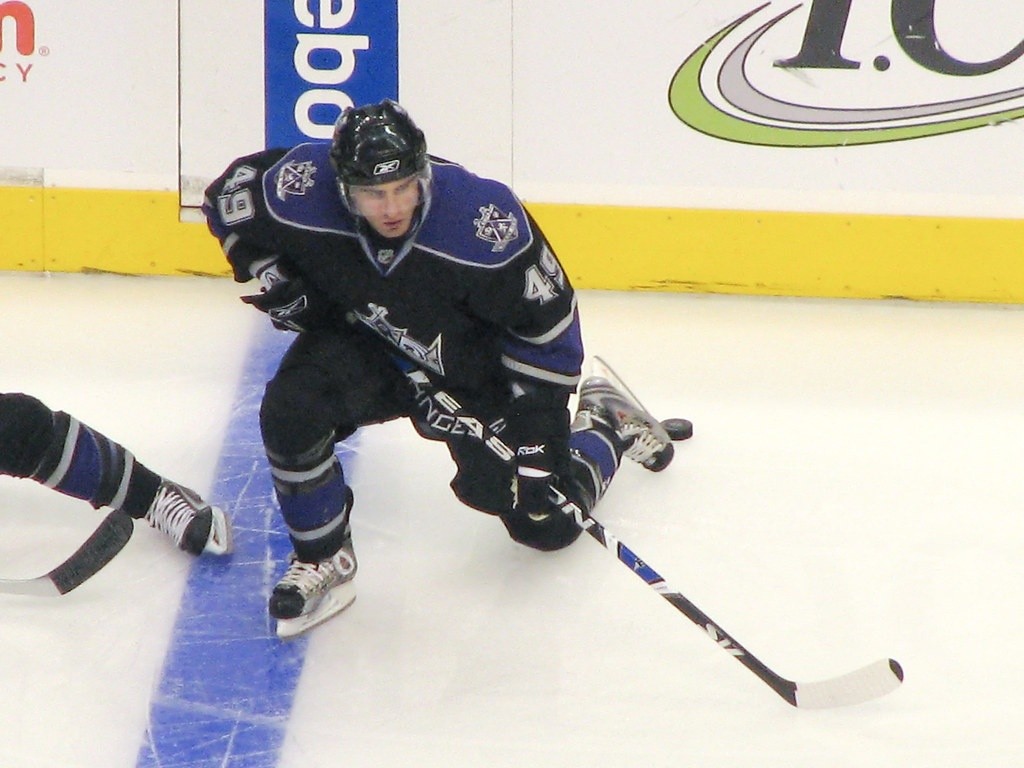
[513,407,574,513]
[238,273,336,333]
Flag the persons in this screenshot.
[202,100,674,639]
[0,392,235,558]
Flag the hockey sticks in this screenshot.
[343,310,905,709]
[0,508,135,598]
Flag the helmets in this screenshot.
[329,98,427,185]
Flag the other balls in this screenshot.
[658,417,694,443]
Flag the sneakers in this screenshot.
[571,354,674,472]
[265,482,359,639]
[140,475,233,557]
